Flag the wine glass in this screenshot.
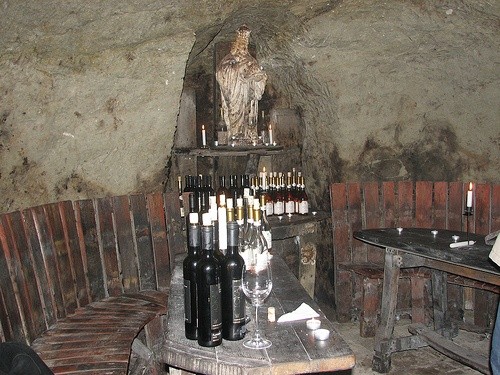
[241,259,272,349]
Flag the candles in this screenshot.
[397,227,403,231]
[268,124,273,144]
[277,215,283,220]
[287,213,293,218]
[431,229,439,234]
[449,239,477,249]
[201,124,207,146]
[312,211,318,215]
[452,234,459,239]
[314,328,331,340]
[262,165,266,189]
[305,316,322,330]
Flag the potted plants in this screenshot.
[466,180,474,207]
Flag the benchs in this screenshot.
[0,189,193,375]
[331,179,500,337]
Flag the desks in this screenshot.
[186,146,286,173]
[266,212,321,299]
[352,226,500,375]
[162,254,355,375]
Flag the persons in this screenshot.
[216,24,268,145]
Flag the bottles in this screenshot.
[183,166,309,348]
